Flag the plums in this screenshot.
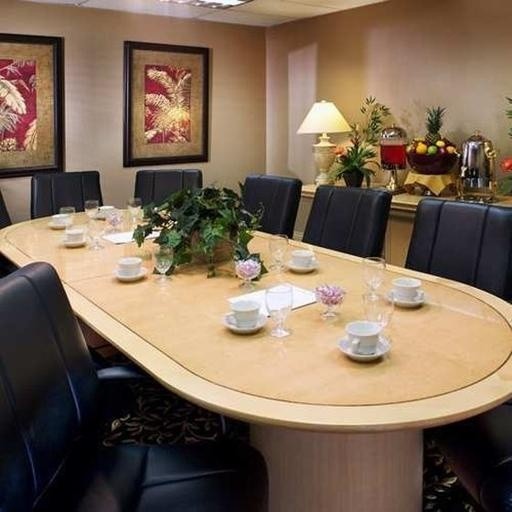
[438,147,447,154]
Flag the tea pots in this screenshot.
[458,129,496,202]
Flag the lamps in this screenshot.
[295,101,351,187]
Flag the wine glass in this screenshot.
[361,257,394,340]
[268,232,290,273]
[148,243,174,289]
[85,196,142,252]
[265,282,294,337]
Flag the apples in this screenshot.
[427,146,438,154]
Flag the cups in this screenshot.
[60,207,84,227]
[51,214,68,224]
[393,277,422,301]
[345,320,380,355]
[118,257,142,275]
[292,250,316,268]
[64,228,84,241]
[227,301,263,328]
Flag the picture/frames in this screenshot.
[122,39,214,165]
[0,32,67,179]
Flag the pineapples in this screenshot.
[424,106,447,144]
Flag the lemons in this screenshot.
[436,141,446,146]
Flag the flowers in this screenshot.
[328,95,391,187]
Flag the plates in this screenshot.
[383,290,427,308]
[113,268,148,281]
[47,222,72,230]
[62,240,87,248]
[287,261,320,273]
[338,337,390,362]
[223,314,267,335]
